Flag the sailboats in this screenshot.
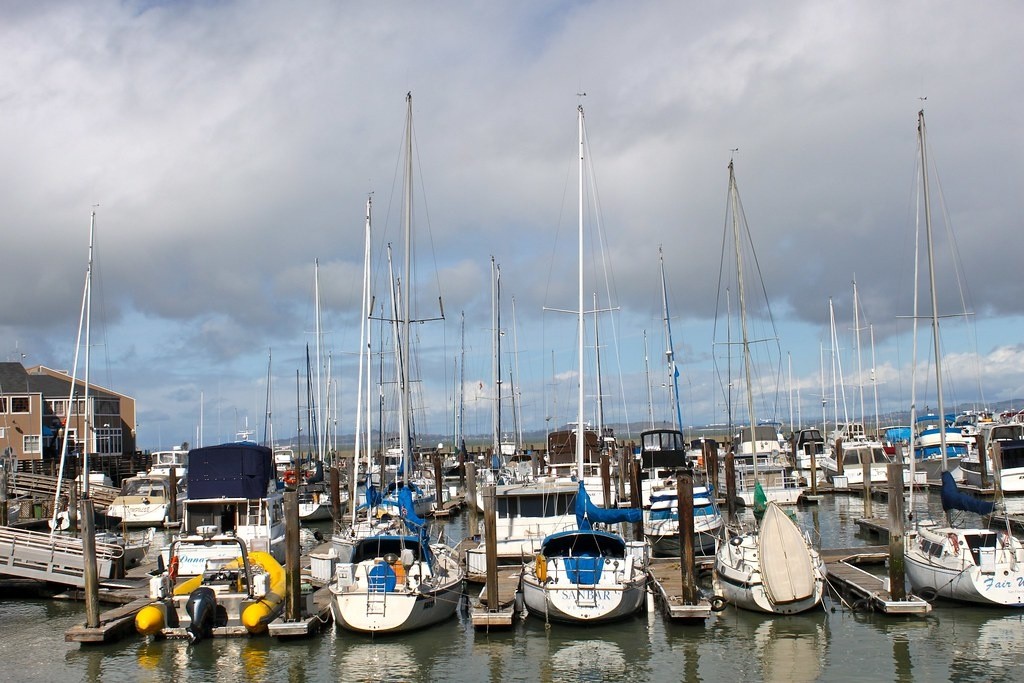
[903,96,1024,605]
[327,92,463,632]
[103,191,1024,575]
[519,92,648,620]
[712,146,826,615]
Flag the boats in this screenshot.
[145,441,285,599]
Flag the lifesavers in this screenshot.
[283,470,298,485]
[169,555,179,581]
[535,554,548,583]
